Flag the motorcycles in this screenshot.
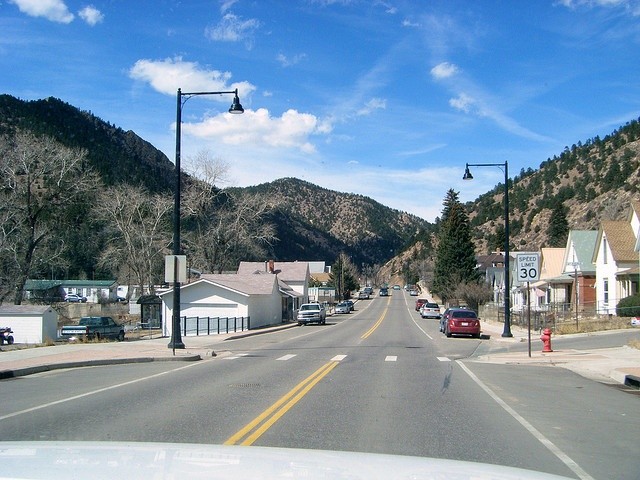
[0,327,14,345]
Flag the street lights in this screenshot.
[463,160,513,336]
[167,88,245,349]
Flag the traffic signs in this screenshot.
[516,253,540,282]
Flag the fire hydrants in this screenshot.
[540,328,553,352]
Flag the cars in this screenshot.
[440,308,480,338]
[358,291,369,299]
[334,303,350,314]
[64,294,87,303]
[416,299,428,312]
[420,303,440,319]
[365,287,373,295]
[379,288,388,296]
[410,289,417,296]
[344,301,354,311]
[393,284,400,290]
[297,303,325,326]
[59,317,124,342]
